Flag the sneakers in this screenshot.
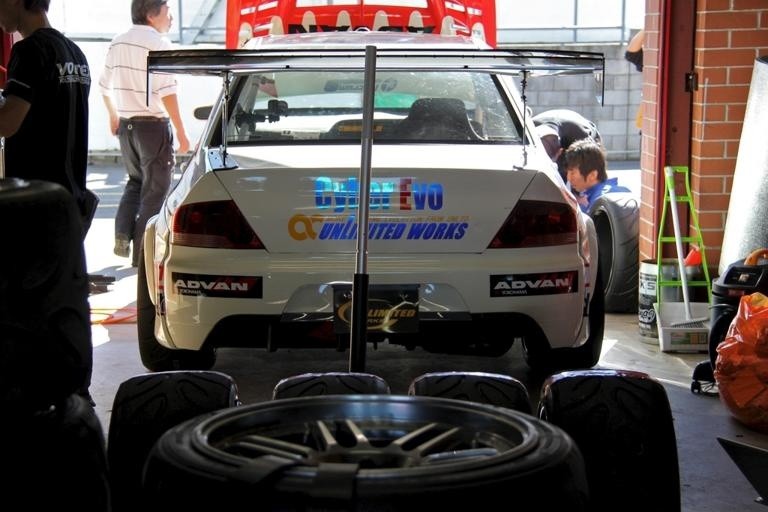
[113,233,130,258]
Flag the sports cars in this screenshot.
[134,25,615,379]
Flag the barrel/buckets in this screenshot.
[637,258,680,345]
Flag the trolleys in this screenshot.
[690,248,767,398]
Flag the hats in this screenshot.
[536,125,560,160]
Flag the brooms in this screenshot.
[662,166,711,329]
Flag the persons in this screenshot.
[100,0,190,268]
[624,30,645,70]
[0,0,97,244]
[533,109,630,213]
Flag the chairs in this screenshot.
[390,98,484,141]
